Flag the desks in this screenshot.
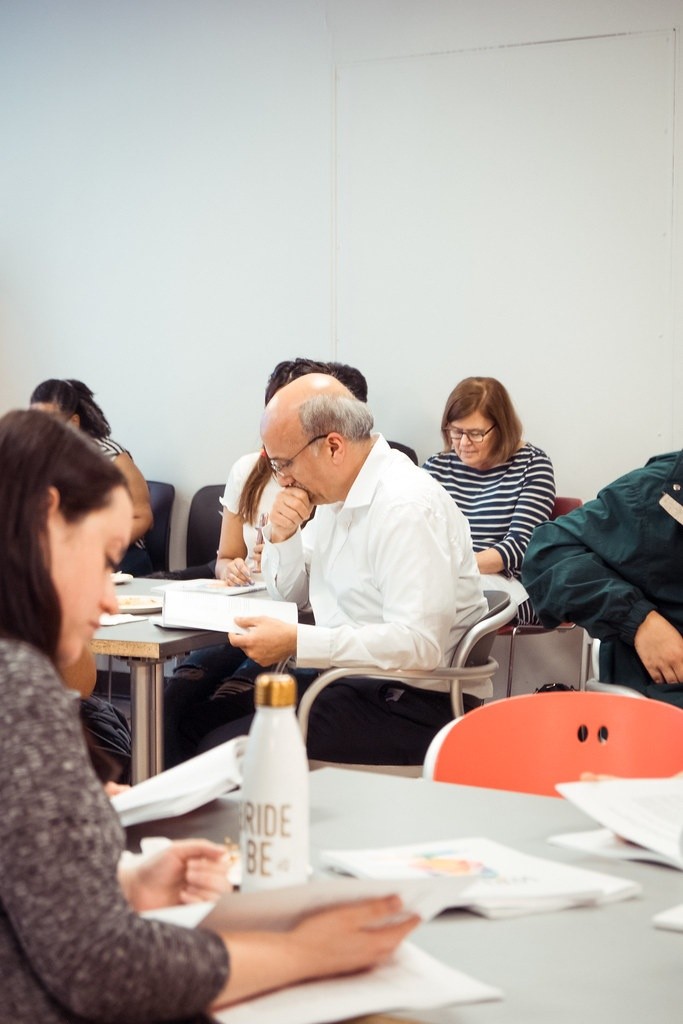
[85,578,313,787]
[126,766,683,1023]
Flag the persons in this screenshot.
[29,379,155,578]
[224,374,494,766]
[0,407,421,1024]
[163,358,368,771]
[422,376,556,581]
[521,448,683,709]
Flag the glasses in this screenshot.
[442,422,497,443]
[271,433,329,477]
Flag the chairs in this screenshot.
[108,481,175,722]
[186,485,225,570]
[274,573,518,746]
[495,497,583,698]
[422,691,683,798]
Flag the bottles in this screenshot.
[239,672,312,893]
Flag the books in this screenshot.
[159,577,267,596]
[142,875,501,1024]
[320,834,639,921]
[150,589,298,636]
[546,772,683,871]
[110,734,248,827]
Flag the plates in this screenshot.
[118,595,162,614]
